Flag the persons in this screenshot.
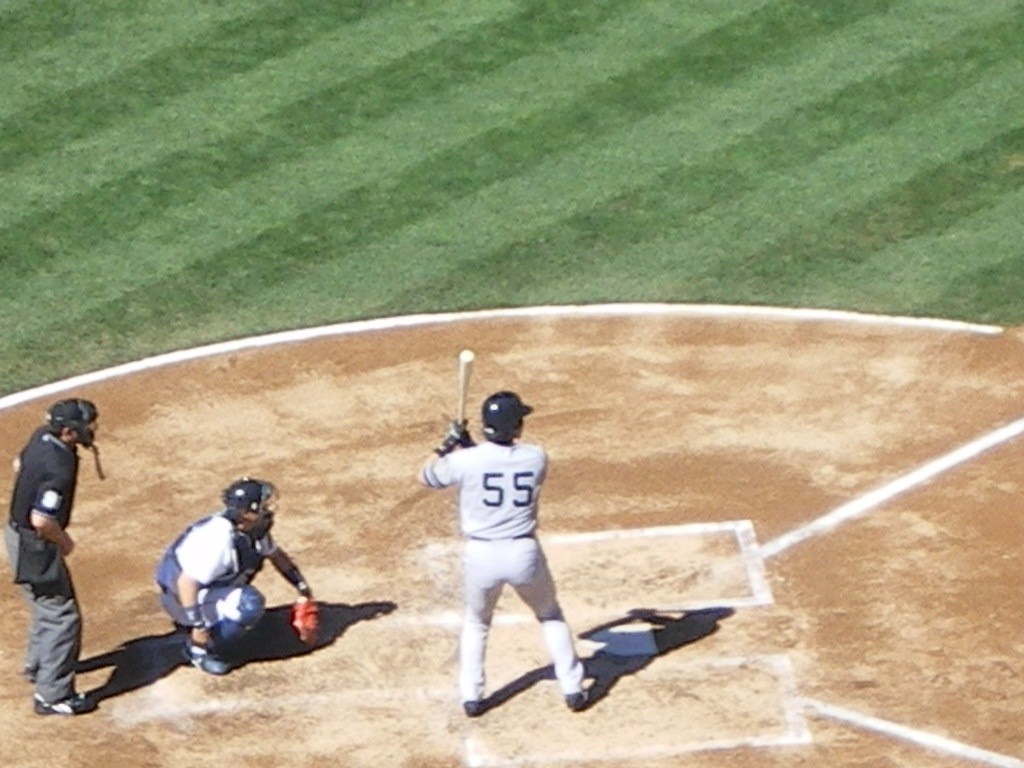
[156,478,320,673]
[7,398,98,715]
[417,391,590,716]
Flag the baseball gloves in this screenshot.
[291,597,323,649]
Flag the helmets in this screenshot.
[47,398,100,450]
[220,476,279,540]
[481,391,534,442]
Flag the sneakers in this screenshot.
[25,667,97,716]
[566,691,594,712]
[181,643,232,676]
[464,701,484,717]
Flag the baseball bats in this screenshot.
[456,349,476,424]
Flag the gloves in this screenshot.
[432,419,476,458]
